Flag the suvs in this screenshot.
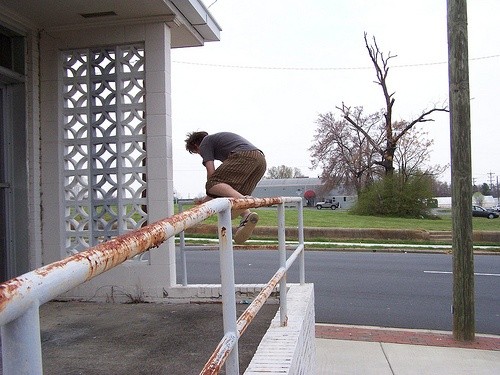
[315,199,340,210]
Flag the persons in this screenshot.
[184,130,267,245]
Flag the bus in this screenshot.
[423,198,439,208]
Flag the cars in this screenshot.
[471,205,499,219]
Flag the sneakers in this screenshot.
[234,212,259,244]
[231,195,252,220]
